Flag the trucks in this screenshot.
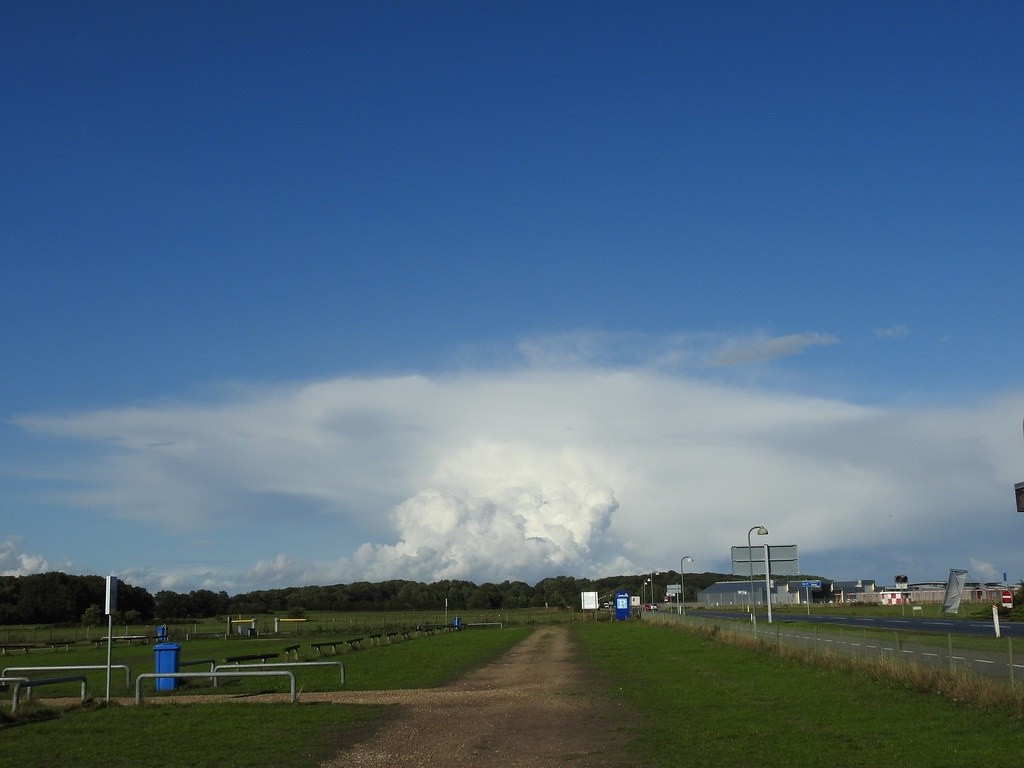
[604,603,609,608]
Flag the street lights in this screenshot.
[644,577,651,603]
[747,526,769,639]
[680,556,693,615]
[650,570,660,604]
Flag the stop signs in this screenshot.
[1002,590,1012,604]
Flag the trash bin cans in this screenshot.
[454,617,461,629]
[155,625,168,642]
[153,642,181,692]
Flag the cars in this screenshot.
[650,603,658,611]
[643,604,650,611]
